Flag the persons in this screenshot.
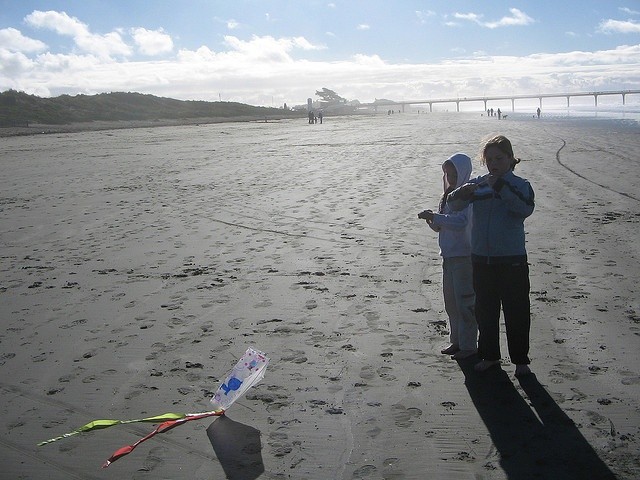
[491,108,493,116]
[447,136,535,377]
[537,108,541,118]
[419,152,478,360]
[311,112,314,124]
[318,112,323,124]
[308,111,312,123]
[498,109,501,120]
[487,109,490,116]
[315,116,317,124]
